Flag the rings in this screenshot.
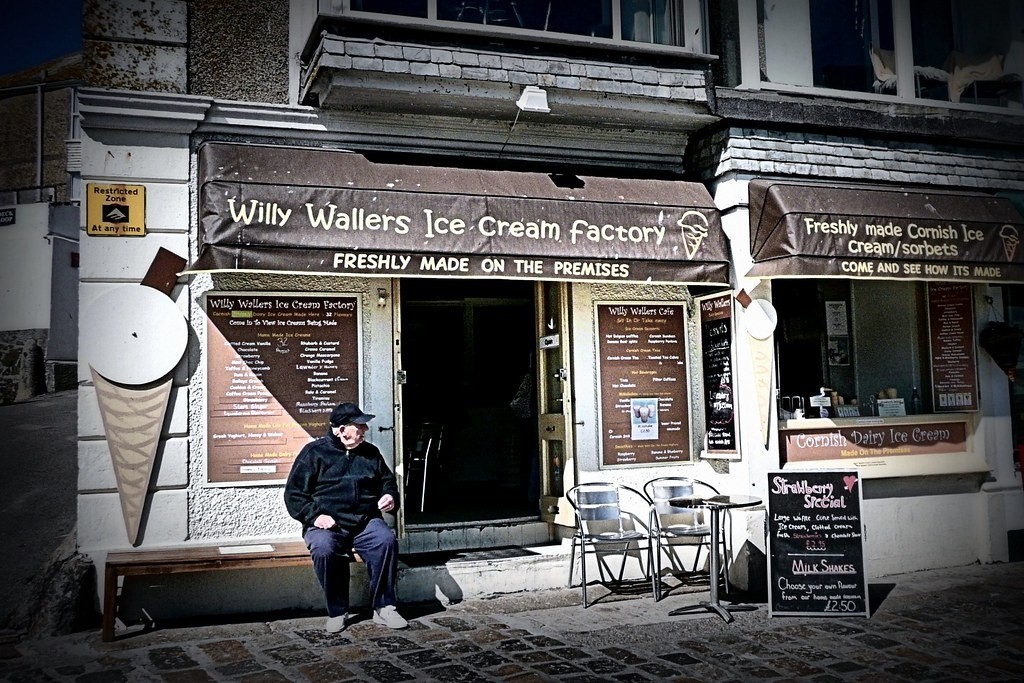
[390,505,392,508]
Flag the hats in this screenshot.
[330,403,376,426]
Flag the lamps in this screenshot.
[516,87,552,113]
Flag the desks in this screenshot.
[668,491,763,623]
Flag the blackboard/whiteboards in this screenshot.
[767,470,870,618]
[699,289,741,460]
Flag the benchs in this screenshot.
[104,542,365,641]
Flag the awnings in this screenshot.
[174,143,734,288]
[745,176,1024,285]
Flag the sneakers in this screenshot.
[373,605,408,628]
[326,611,348,632]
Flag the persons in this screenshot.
[284,403,407,633]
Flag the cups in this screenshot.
[648,405,655,418]
[633,405,641,418]
[639,407,649,423]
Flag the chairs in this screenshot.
[644,477,730,600]
[869,43,1004,105]
[566,482,657,609]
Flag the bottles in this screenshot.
[911,389,920,415]
